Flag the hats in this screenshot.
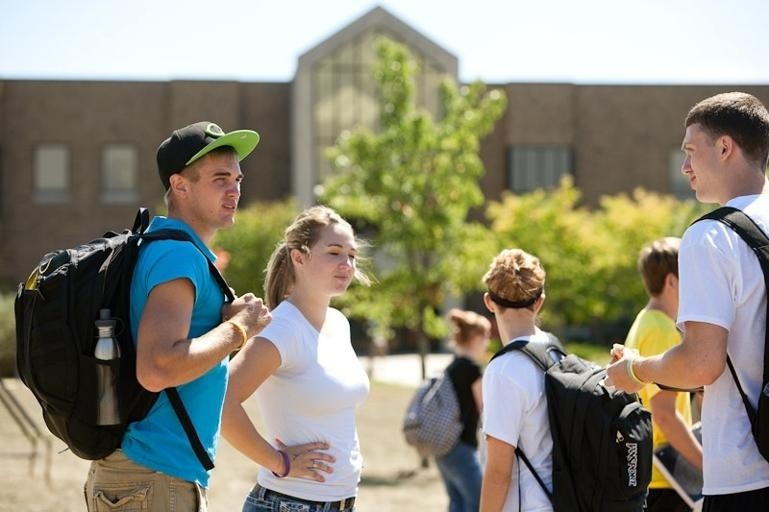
[155,120,260,191]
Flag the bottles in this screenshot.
[94,309,121,362]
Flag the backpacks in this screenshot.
[401,359,468,468]
[688,206,769,464]
[13,206,236,472]
[484,331,653,512]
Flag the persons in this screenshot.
[83,121,271,512]
[217,203,370,512]
[478,247,564,512]
[434,309,491,512]
[622,236,703,512]
[603,89,769,512]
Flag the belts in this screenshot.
[334,497,355,510]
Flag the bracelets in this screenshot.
[225,317,248,351]
[626,357,650,388]
[272,450,291,478]
